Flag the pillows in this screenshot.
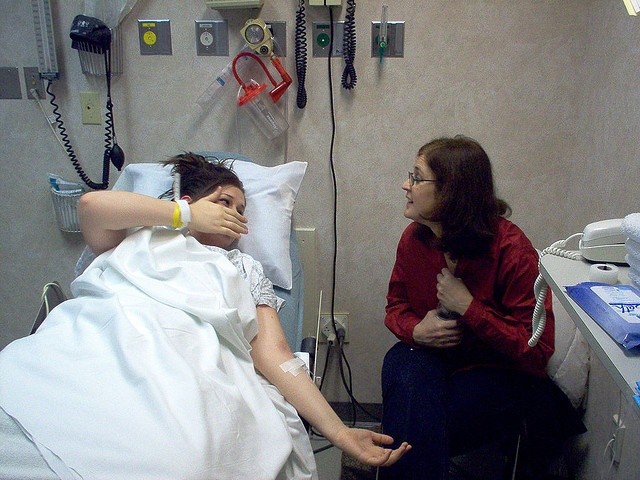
[128,159,309,291]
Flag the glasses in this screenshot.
[407,172,436,186]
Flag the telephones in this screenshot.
[528,216,628,347]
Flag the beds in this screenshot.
[0,218,308,480]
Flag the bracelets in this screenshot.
[171,200,190,231]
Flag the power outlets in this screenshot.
[314,312,350,345]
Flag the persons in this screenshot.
[1,154,412,480]
[383,136,557,478]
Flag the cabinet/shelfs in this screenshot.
[537,252,640,480]
